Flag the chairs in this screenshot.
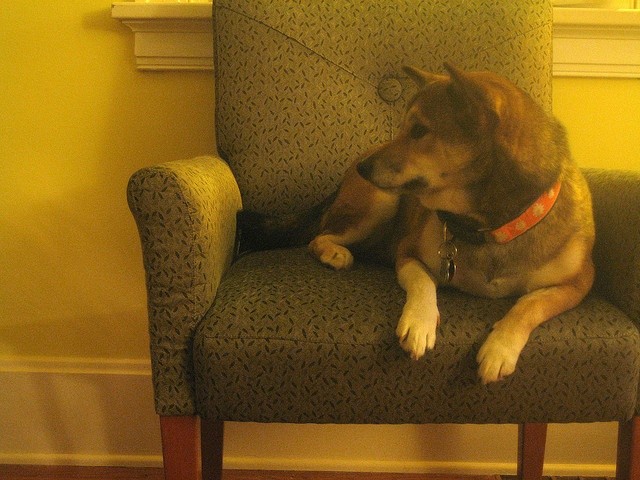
[125,0,638,479]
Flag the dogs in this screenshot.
[235,59,597,386]
